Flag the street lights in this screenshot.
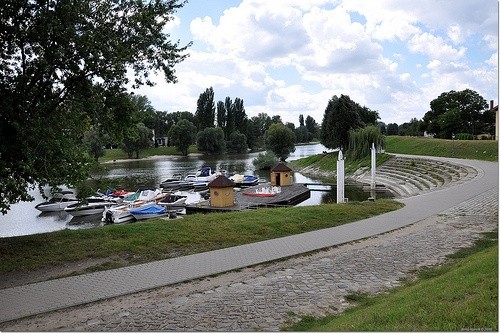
[451,131,456,158]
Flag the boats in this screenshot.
[34,183,214,224]
[159,166,259,192]
